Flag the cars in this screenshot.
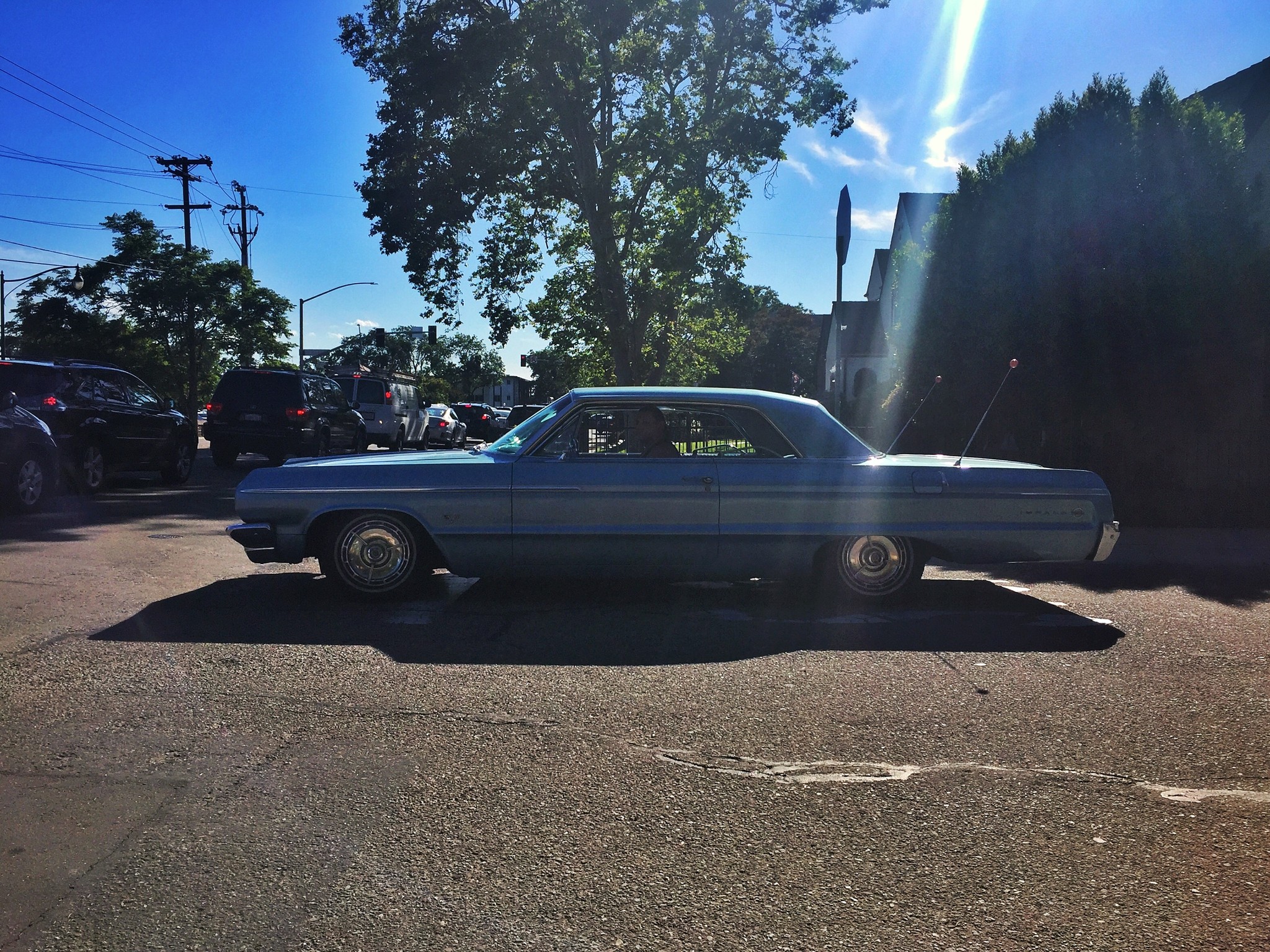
[425,403,467,450]
[587,412,621,434]
[0,390,59,515]
[226,385,1122,610]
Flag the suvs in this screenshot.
[450,402,501,443]
[202,366,368,468]
[327,364,433,454]
[490,403,550,435]
[0,357,199,496]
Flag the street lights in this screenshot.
[0,263,85,362]
[299,282,378,373]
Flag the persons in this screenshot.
[634,406,681,457]
